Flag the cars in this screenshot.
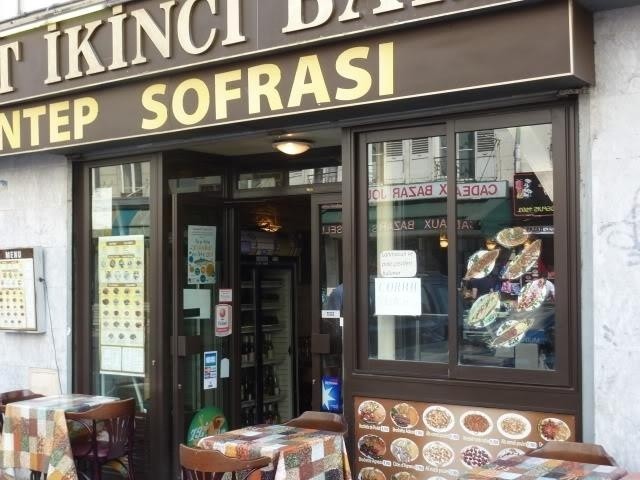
[321,270,470,382]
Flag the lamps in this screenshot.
[271,139,315,156]
[440,235,448,247]
[486,239,497,251]
[257,214,283,232]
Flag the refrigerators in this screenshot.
[237,254,299,428]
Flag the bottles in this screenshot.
[240,336,255,366]
[262,334,273,362]
[241,403,281,428]
[240,372,252,403]
[263,366,280,398]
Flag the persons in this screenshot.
[328,260,375,324]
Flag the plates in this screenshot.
[496,227,528,248]
[358,400,571,442]
[495,319,525,348]
[357,435,525,480]
[517,281,547,312]
[466,250,496,280]
[469,292,500,328]
[505,245,541,280]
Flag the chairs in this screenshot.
[179,443,272,480]
[278,410,349,440]
[65,397,136,480]
[525,441,619,466]
[0,389,48,480]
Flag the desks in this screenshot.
[456,454,640,480]
[0,393,129,480]
[195,423,353,480]
